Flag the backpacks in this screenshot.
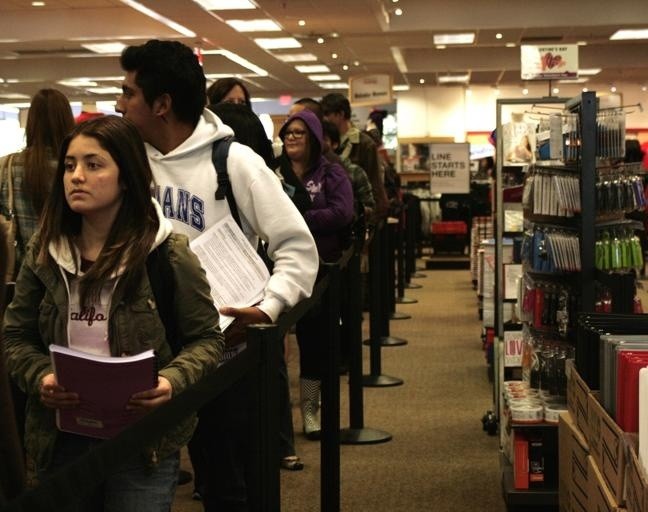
[208,102,276,230]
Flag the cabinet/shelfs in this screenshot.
[503,91,645,511]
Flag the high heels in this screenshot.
[282,456,303,471]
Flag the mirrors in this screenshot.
[500,104,568,332]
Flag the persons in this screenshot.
[284,97,321,121]
[272,110,354,439]
[318,118,378,378]
[318,91,385,368]
[1,115,227,511]
[115,37,319,511]
[1,87,76,283]
[477,157,495,175]
[276,339,306,472]
[207,77,253,111]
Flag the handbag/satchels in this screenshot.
[0,213,17,301]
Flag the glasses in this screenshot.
[282,129,308,139]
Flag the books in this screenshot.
[48,343,160,439]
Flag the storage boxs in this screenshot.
[586,456,616,512]
[566,358,591,445]
[626,433,648,512]
[558,414,589,512]
[587,393,626,507]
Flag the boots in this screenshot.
[300,377,321,440]
